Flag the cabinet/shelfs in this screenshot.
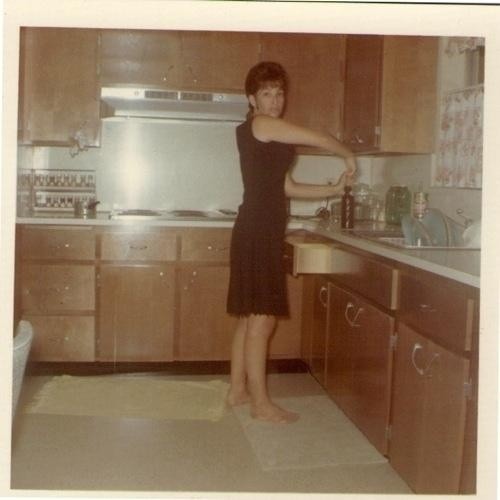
[321,246,400,462]
[19,225,102,369]
[97,29,262,94]
[388,265,474,493]
[283,229,332,389]
[19,27,103,151]
[259,32,347,157]
[345,35,439,155]
[95,229,234,365]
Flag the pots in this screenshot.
[74,200,100,218]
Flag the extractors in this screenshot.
[101,87,250,122]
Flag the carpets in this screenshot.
[22,373,231,423]
[234,394,392,470]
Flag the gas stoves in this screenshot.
[109,208,230,222]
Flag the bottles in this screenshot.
[35,173,95,188]
[36,195,94,208]
[341,182,430,230]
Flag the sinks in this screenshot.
[347,228,480,251]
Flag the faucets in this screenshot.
[424,205,475,231]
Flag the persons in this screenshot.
[226,60,359,427]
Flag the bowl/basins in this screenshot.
[403,208,467,248]
